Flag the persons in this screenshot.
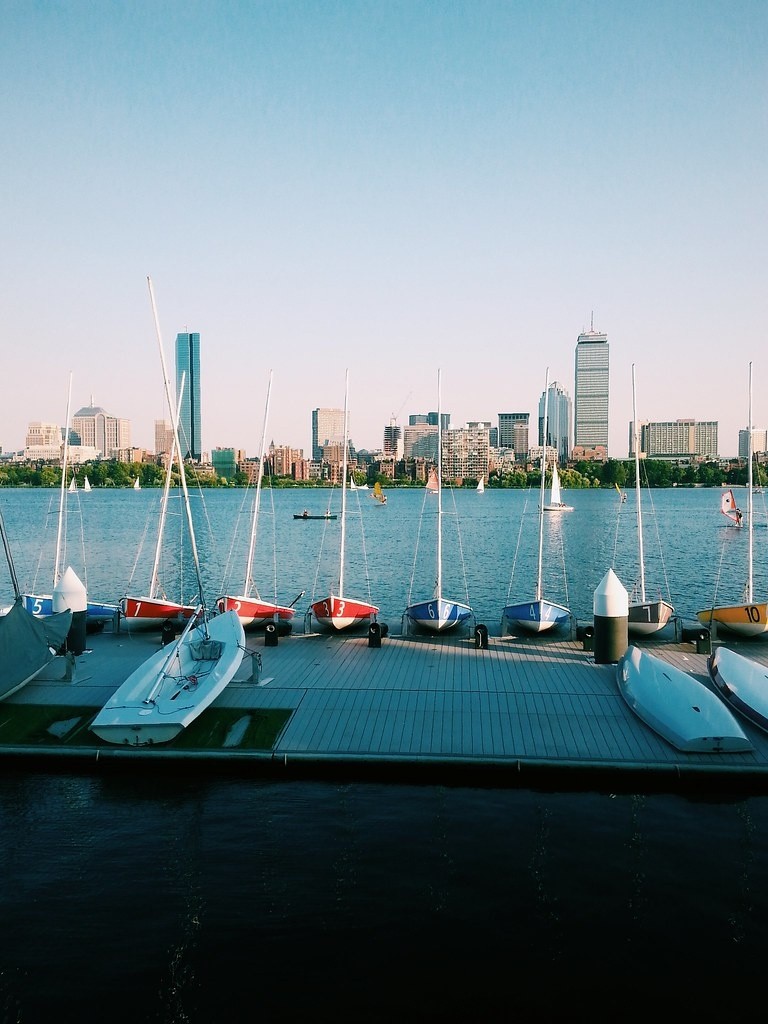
[558,501,565,508]
[383,494,387,504]
[736,508,743,527]
[302,509,309,517]
[325,508,331,516]
[623,492,627,503]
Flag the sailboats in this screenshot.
[213,366,296,628]
[85,275,249,749]
[404,368,473,631]
[538,449,574,511]
[696,361,768,638]
[307,367,380,631]
[502,366,572,633]
[426,467,438,493]
[20,370,123,621]
[119,370,210,627]
[476,476,485,493]
[350,476,369,490]
[611,363,675,635]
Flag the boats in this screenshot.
[81,475,92,492]
[615,645,756,752]
[706,646,768,732]
[292,514,337,519]
[752,487,765,494]
[67,477,79,494]
[133,476,142,490]
